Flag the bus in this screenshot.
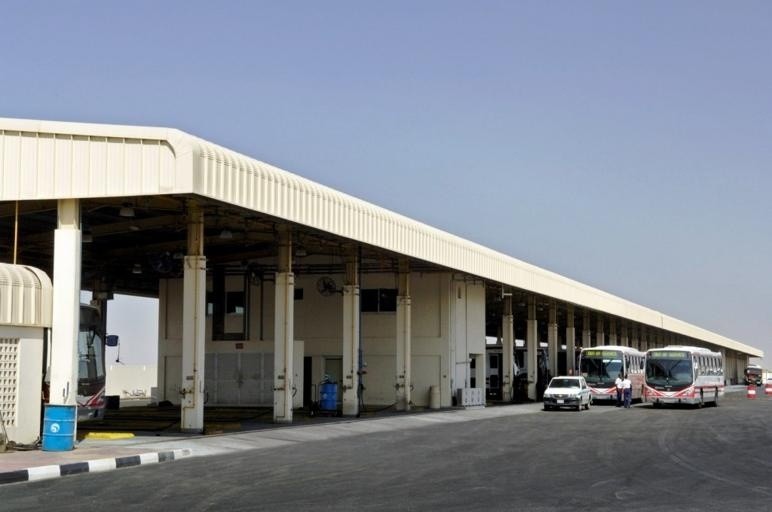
[41,302,106,422]
[642,344,724,408]
[744,365,762,385]
[577,345,647,403]
[471,345,548,394]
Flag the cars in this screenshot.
[543,375,592,410]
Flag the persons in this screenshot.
[615,374,623,407]
[623,374,632,408]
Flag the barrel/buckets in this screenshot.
[318,383,338,411]
[41,403,77,452]
[429,384,441,409]
